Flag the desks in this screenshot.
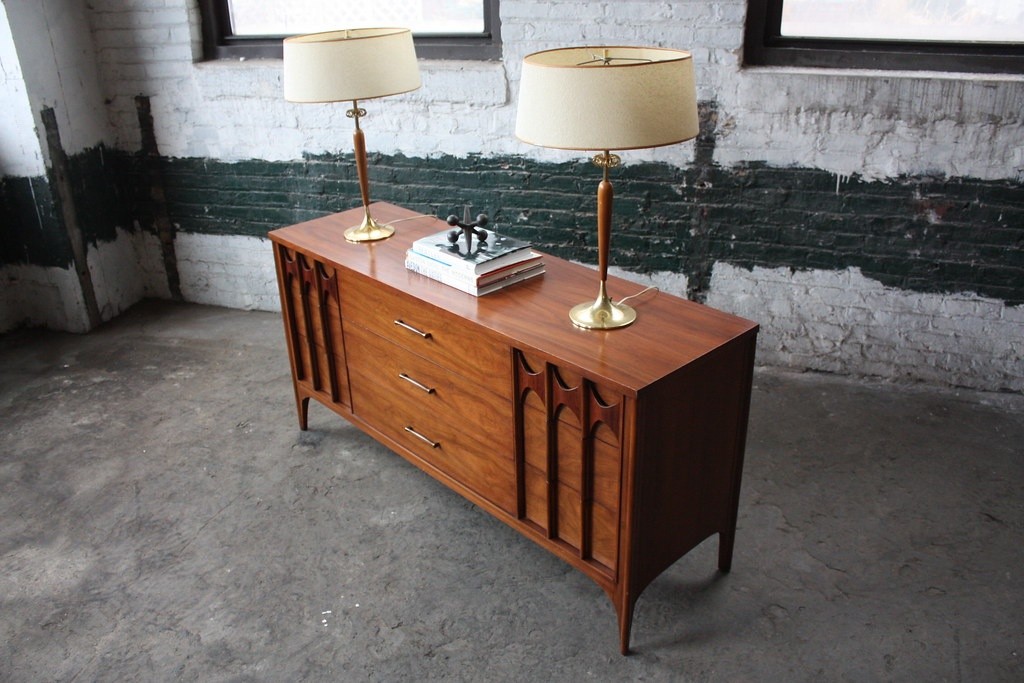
[268,201,760,656]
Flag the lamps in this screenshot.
[514,45,699,330]
[282,28,423,242]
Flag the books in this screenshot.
[403,224,546,298]
